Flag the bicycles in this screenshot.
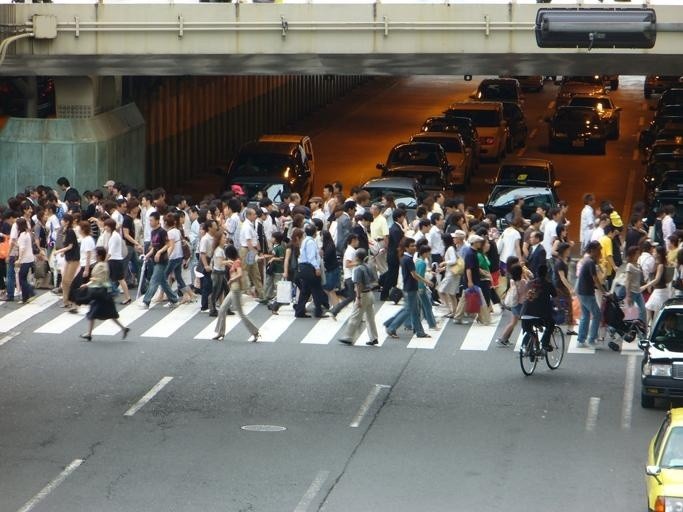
[518,312,567,375]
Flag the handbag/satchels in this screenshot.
[276,275,293,304]
[0,235,11,259]
[447,256,465,275]
[30,231,41,255]
[230,270,252,291]
[649,266,675,285]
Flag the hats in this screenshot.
[102,180,115,188]
[450,229,466,239]
[644,238,660,247]
[468,234,485,244]
[308,196,323,203]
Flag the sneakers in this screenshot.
[587,342,602,350]
[339,338,353,345]
[382,300,456,331]
[576,340,591,349]
[51,286,78,312]
[138,292,199,309]
[366,339,378,345]
[258,298,366,323]
[0,294,14,301]
[566,329,578,336]
[528,351,539,362]
[80,334,92,342]
[495,336,513,347]
[453,317,469,324]
[386,328,401,338]
[121,327,130,340]
[542,341,554,352]
[416,332,432,338]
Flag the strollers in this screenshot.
[602,290,645,352]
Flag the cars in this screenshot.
[559,75,619,92]
[641,297,683,407]
[643,404,682,511]
[553,81,607,113]
[442,101,513,164]
[483,157,562,195]
[639,75,683,231]
[350,176,426,210]
[477,185,564,225]
[502,102,529,150]
[375,141,455,197]
[498,75,546,93]
[467,77,522,106]
[420,115,489,167]
[543,105,608,157]
[224,132,318,208]
[407,130,474,193]
[567,93,624,141]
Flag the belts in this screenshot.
[361,289,371,293]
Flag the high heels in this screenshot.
[212,333,225,340]
[253,331,261,342]
[120,297,132,305]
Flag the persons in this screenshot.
[196,182,445,345]
[1,176,200,342]
[438,194,682,350]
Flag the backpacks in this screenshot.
[504,281,520,308]
[181,240,191,260]
